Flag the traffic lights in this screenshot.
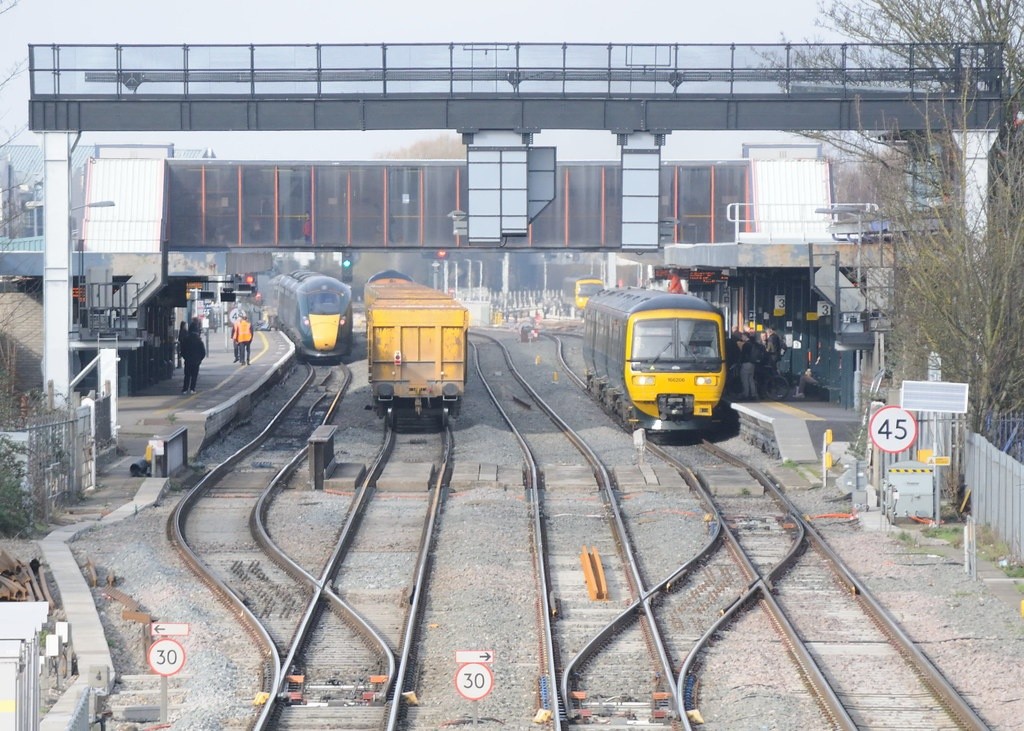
[341,252,353,284]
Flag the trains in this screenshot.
[582,284,727,438]
[361,277,471,427]
[269,268,354,364]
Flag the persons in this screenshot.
[177,316,202,369]
[180,324,205,395]
[231,312,252,365]
[303,211,317,244]
[724,322,832,405]
[22,182,44,237]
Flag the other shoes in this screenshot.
[793,392,805,398]
[191,390,196,394]
[182,391,187,394]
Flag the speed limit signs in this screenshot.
[868,405,919,454]
[456,664,493,699]
[148,639,186,676]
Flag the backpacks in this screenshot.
[749,343,762,364]
